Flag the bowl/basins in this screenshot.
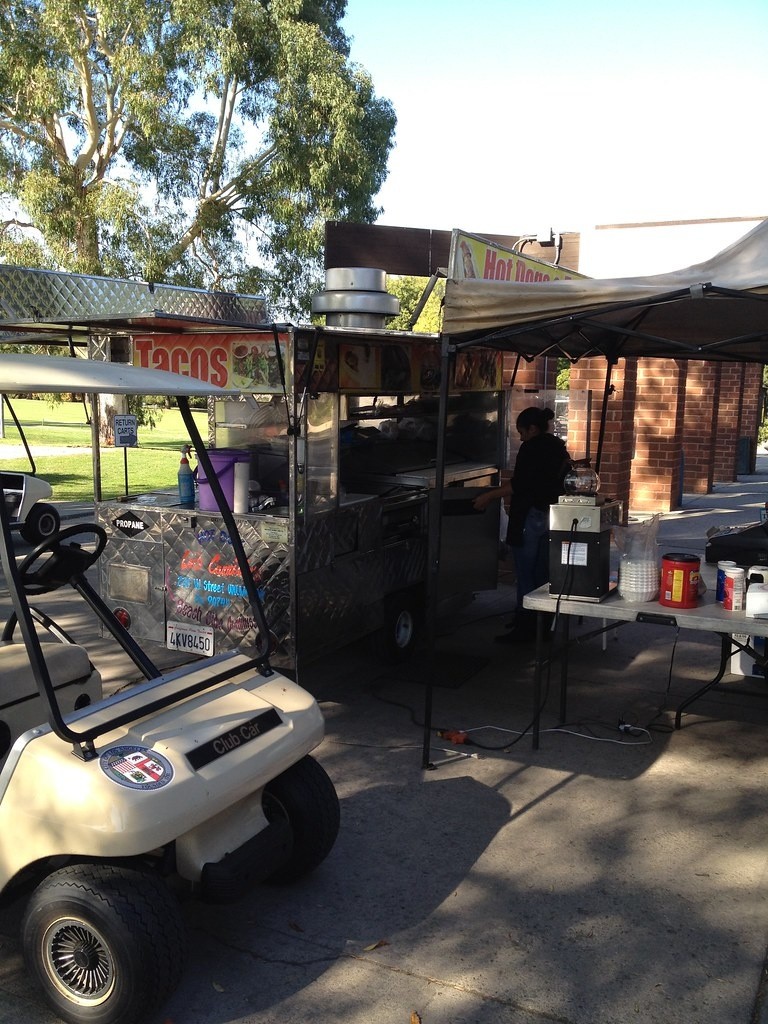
[617,559,660,603]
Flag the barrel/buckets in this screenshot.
[193,448,247,512]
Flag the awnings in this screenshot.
[0,309,294,432]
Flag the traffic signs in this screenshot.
[114,414,139,448]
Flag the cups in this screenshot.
[233,462,249,514]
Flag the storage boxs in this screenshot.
[731,633,765,679]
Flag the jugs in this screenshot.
[563,458,601,496]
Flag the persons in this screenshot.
[473,406,571,640]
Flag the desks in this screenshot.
[523,572,768,750]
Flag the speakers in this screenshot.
[736,435,753,475]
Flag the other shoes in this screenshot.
[511,626,536,641]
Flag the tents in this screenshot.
[423,217,768,768]
